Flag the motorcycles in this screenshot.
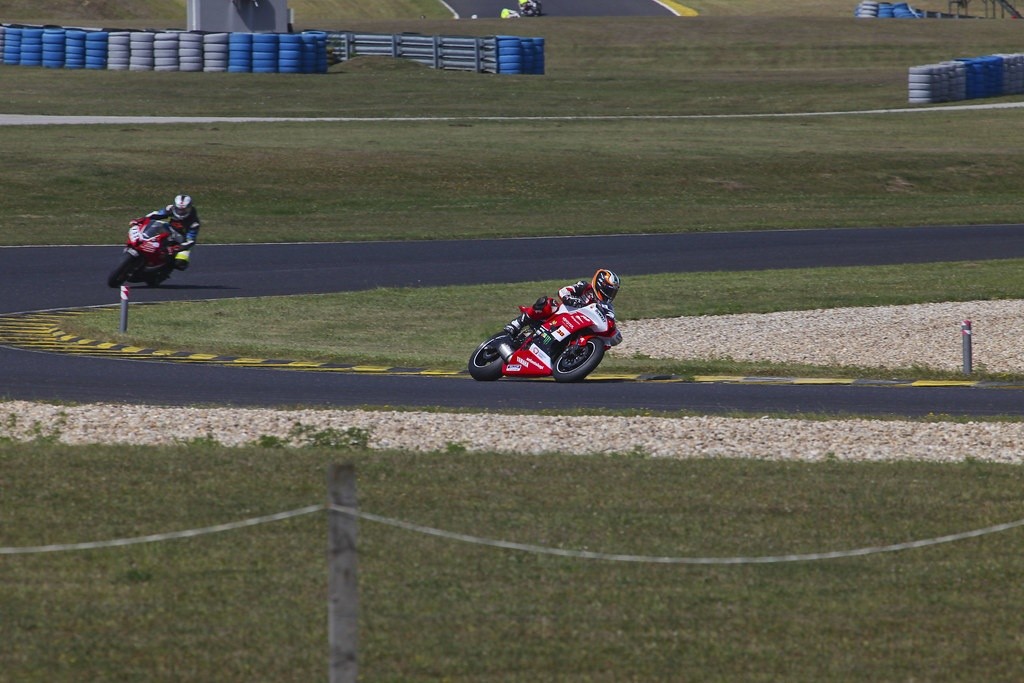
[106,217,188,289]
[468,297,623,384]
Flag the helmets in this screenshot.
[592,269,621,304]
[172,194,193,220]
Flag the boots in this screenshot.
[503,312,533,338]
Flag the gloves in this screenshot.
[563,294,582,307]
[128,218,145,228]
[165,245,184,255]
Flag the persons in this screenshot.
[137,195,200,271]
[502,267,621,339]
